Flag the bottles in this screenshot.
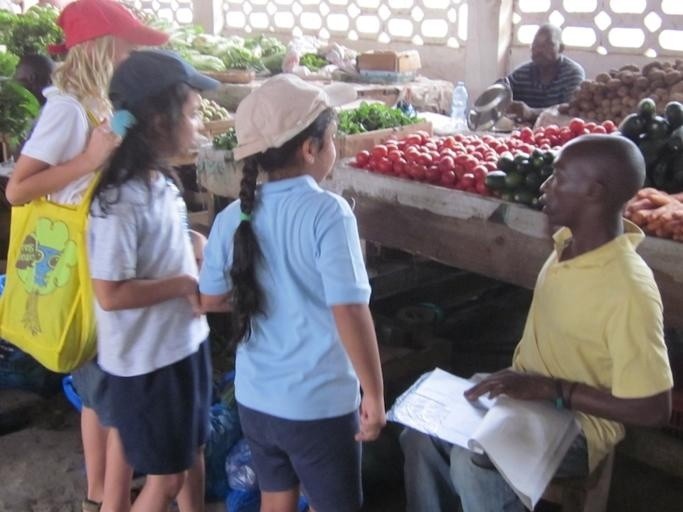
[450,80,468,137]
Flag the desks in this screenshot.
[200,76,454,122]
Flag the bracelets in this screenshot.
[555,376,577,408]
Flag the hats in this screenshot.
[46,1,169,50]
[234,73,359,159]
[108,48,219,110]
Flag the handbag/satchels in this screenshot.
[0,192,96,374]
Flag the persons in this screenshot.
[12,53,53,105]
[397,133,675,511]
[84,50,217,511]
[194,73,387,511]
[3,2,167,512]
[496,24,584,127]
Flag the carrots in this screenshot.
[623,187,683,241]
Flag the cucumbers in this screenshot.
[484,97,683,210]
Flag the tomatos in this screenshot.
[350,117,618,196]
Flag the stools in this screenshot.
[471,419,617,512]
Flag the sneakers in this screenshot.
[82,484,138,512]
